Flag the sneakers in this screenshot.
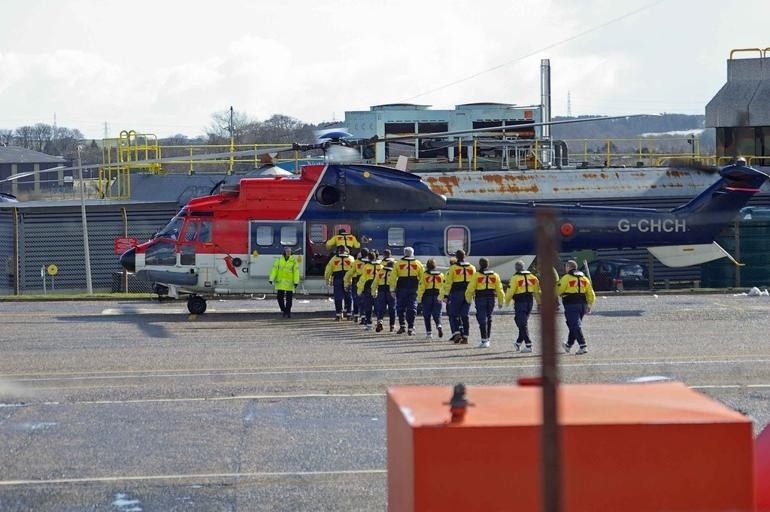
[376,323,382,332]
[514,342,521,351]
[461,338,468,343]
[454,335,462,344]
[480,338,490,348]
[335,314,372,325]
[576,344,588,355]
[562,342,571,352]
[521,343,532,353]
[390,326,416,335]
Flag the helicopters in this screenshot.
[0,114,770,314]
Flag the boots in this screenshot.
[425,332,432,339]
[437,324,442,338]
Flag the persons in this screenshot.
[464,258,505,348]
[554,261,595,354]
[268,247,300,318]
[504,261,539,351]
[325,229,477,343]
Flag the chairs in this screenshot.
[308,240,329,274]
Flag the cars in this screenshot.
[578,257,649,292]
